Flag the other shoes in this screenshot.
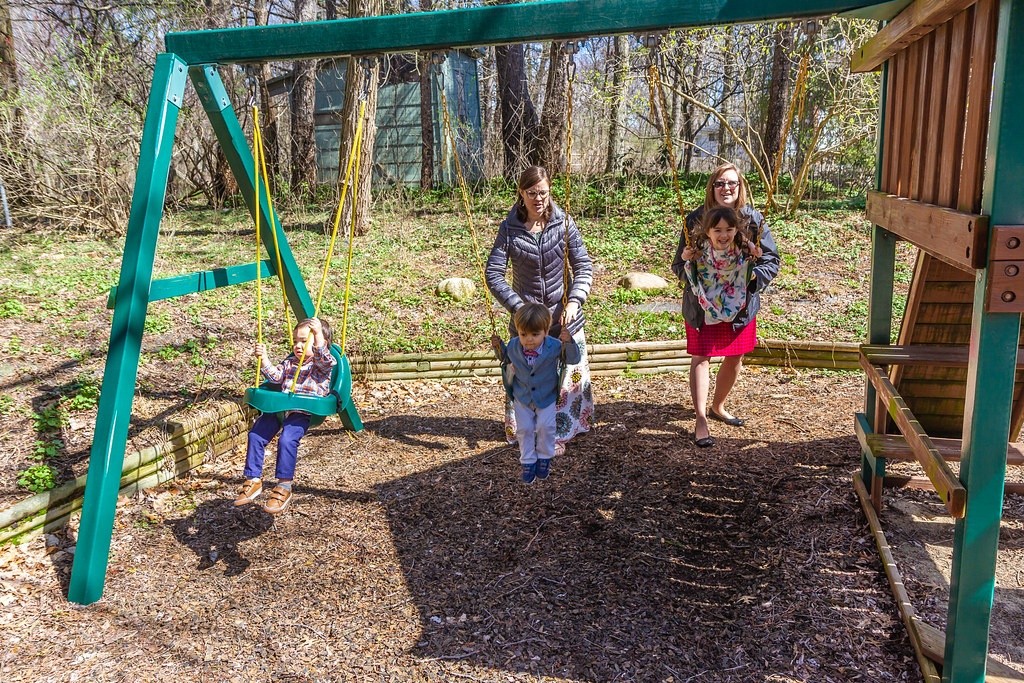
[554,443,567,457]
[694,428,714,447]
[708,407,744,426]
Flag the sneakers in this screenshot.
[233,479,263,506]
[263,486,293,513]
[535,458,550,481]
[522,463,537,485]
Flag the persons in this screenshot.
[669,163,782,447]
[233,317,338,513]
[489,303,581,486]
[484,165,595,457]
[683,205,764,326]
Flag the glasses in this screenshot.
[712,181,741,189]
[524,189,550,199]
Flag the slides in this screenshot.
[881,243,1024,446]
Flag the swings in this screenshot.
[237,55,352,429]
[632,14,832,293]
[423,38,583,405]
